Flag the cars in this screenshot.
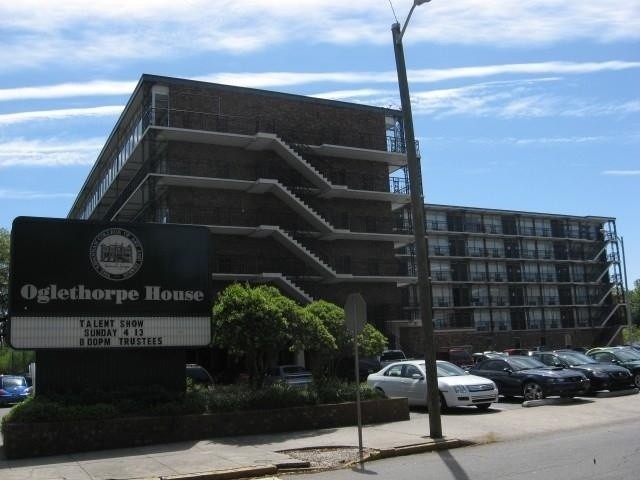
[0,374,29,407]
[186,363,215,387]
[275,365,314,385]
[366,344,640,413]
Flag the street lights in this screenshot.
[387,0,443,438]
